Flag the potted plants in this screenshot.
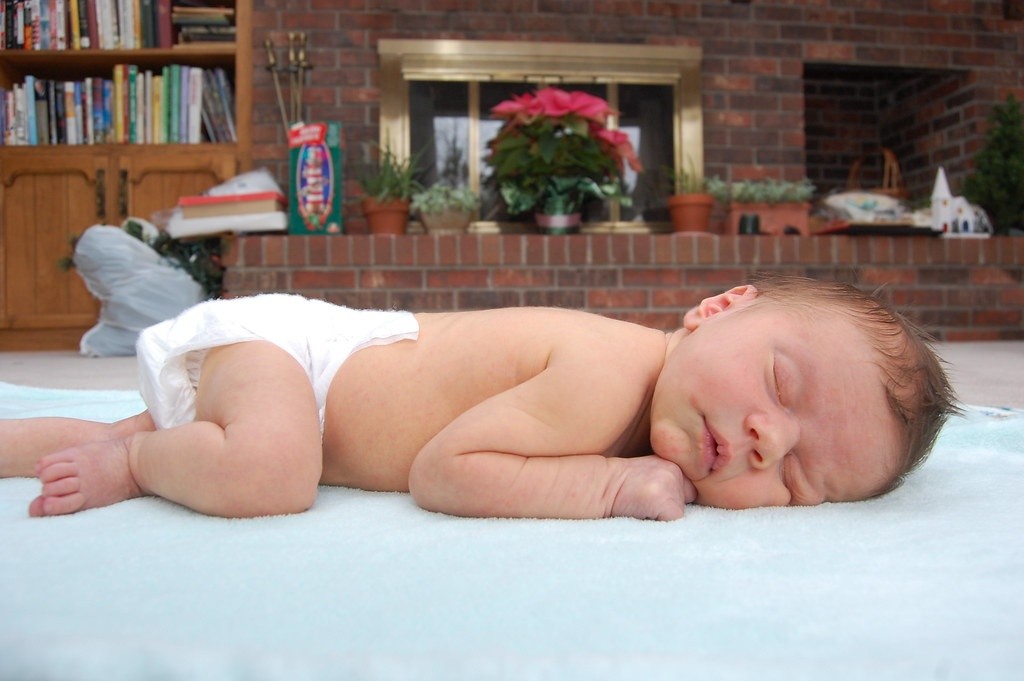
[410,184,482,233]
[659,153,714,232]
[710,175,814,234]
[346,127,430,234]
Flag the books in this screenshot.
[0,0,239,146]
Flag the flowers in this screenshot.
[478,88,643,213]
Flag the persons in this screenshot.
[2,279,953,522]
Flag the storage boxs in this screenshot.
[288,120,345,236]
[179,192,285,219]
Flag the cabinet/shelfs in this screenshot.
[0,0,252,353]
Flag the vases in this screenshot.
[531,197,583,236]
